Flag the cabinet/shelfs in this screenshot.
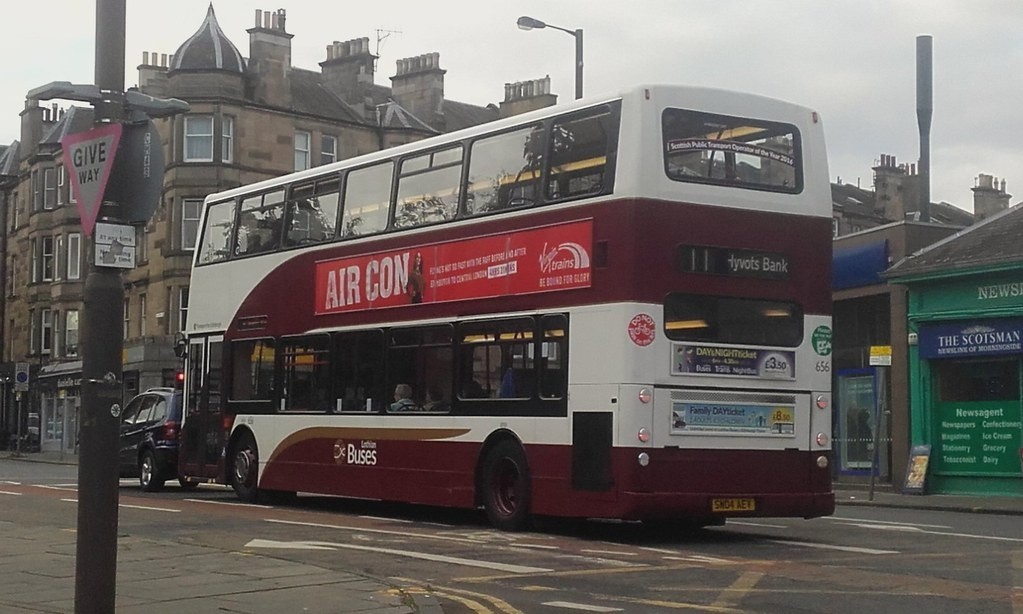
[836,366,889,477]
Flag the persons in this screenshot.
[390,384,423,411]
[405,251,426,304]
[419,386,451,412]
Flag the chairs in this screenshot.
[395,369,568,414]
[213,192,567,274]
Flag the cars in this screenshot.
[123,388,195,491]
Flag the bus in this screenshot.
[174,76,837,540]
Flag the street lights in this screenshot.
[515,12,587,98]
